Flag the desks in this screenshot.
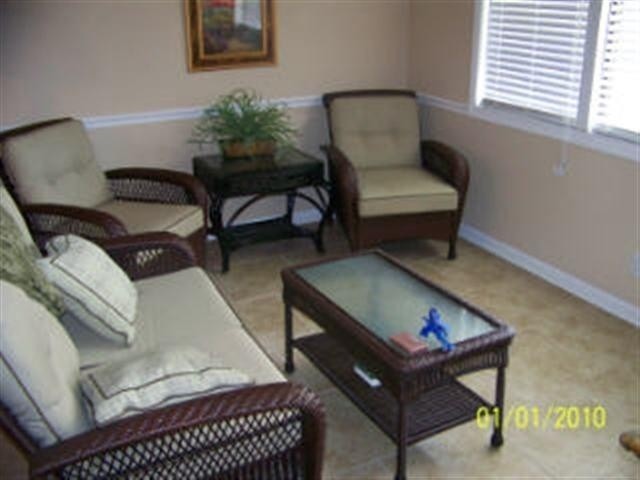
[193,148,335,273]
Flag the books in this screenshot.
[390,330,429,355]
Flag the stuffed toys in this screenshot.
[419,306,454,353]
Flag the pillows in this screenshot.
[81,342,256,423]
[32,233,140,347]
[0,186,68,316]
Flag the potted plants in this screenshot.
[186,82,297,161]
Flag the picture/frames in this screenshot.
[182,0,278,73]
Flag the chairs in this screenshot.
[0,116,210,280]
[319,86,471,260]
[3,229,325,478]
